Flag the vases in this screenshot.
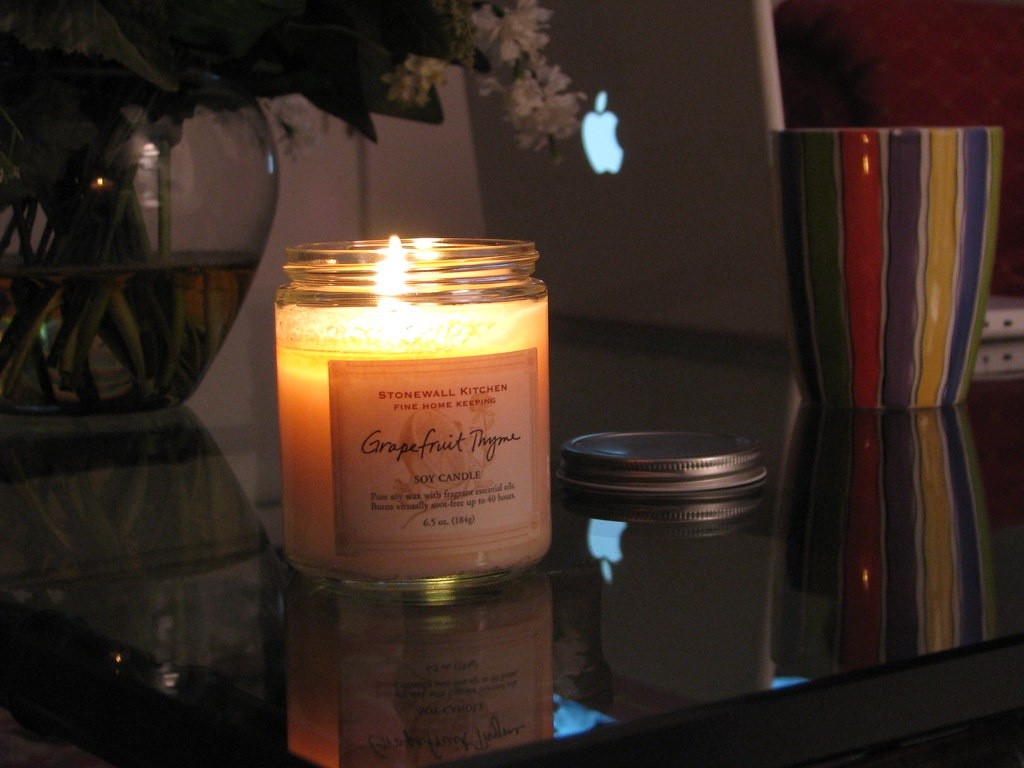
[0,47,279,434]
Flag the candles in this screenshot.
[274,237,551,604]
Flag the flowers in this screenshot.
[0,0,583,398]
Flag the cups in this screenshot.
[768,129,1002,410]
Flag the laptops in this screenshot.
[463,0,1024,343]
[547,341,1024,704]
